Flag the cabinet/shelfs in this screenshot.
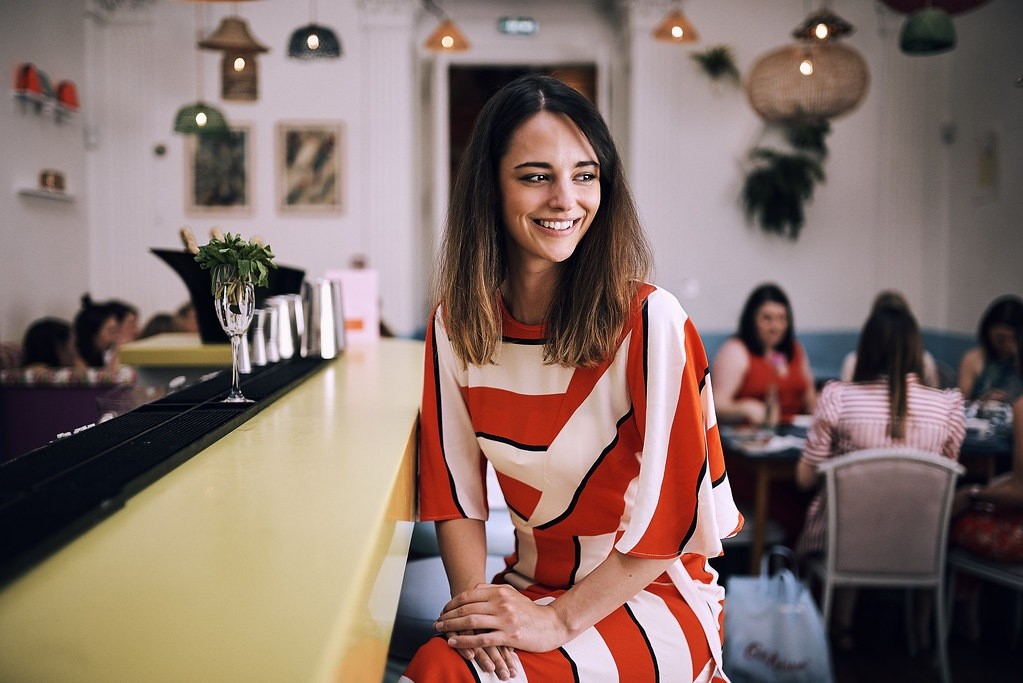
[13,92,84,203]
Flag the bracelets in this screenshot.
[970,482,993,511]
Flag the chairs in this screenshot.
[806,443,1023,683]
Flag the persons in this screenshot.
[953,394,1023,643]
[19,302,199,368]
[398,77,745,683]
[713,284,818,564]
[957,294,1023,476]
[794,310,966,655]
[839,292,940,388]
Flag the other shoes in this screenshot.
[966,615,982,644]
[911,620,933,650]
[832,622,857,657]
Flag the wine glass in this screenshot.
[215,282,254,402]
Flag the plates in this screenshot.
[723,413,820,456]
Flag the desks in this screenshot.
[722,418,1016,579]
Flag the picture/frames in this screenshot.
[182,119,257,218]
[274,119,345,218]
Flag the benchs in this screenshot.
[0,340,135,463]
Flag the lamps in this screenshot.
[171,0,228,132]
[287,0,339,59]
[652,0,697,42]
[899,1,957,56]
[196,0,267,51]
[425,20,466,50]
[791,0,854,40]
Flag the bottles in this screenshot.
[238,294,302,374]
[339,257,380,345]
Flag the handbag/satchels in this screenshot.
[723,545,834,683]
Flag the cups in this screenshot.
[960,398,1009,440]
[301,278,344,358]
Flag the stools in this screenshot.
[381,458,517,683]
